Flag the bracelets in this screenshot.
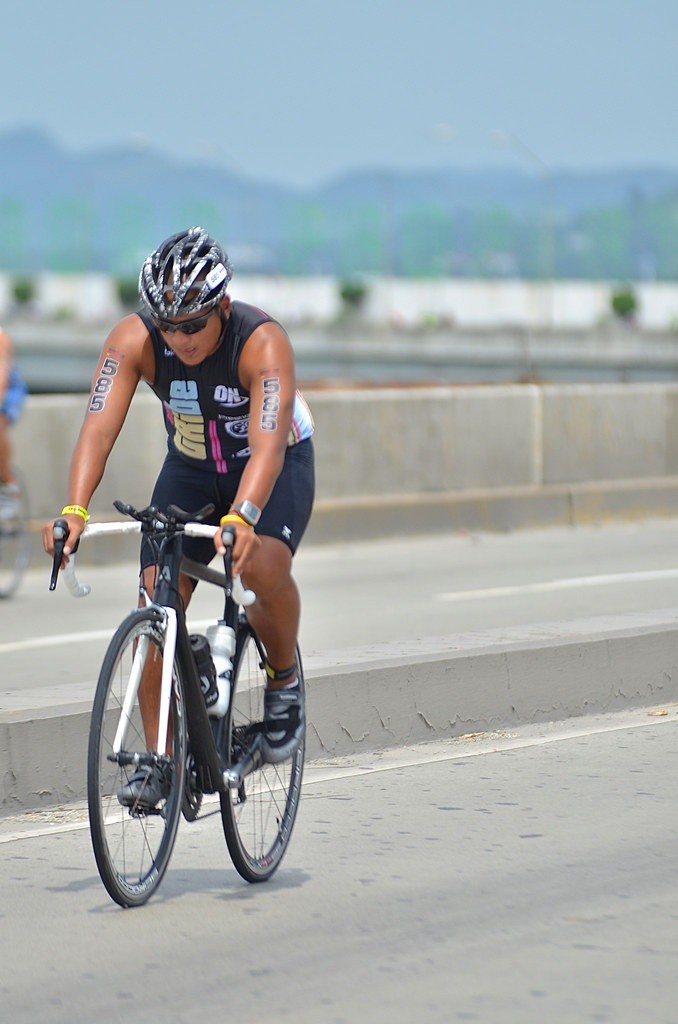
[220,515,255,531]
[61,504,90,523]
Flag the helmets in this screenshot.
[137,227,235,318]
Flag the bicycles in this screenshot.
[48,499,309,910]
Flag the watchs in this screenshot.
[232,499,262,524]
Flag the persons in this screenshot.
[41,227,315,807]
[0,331,28,518]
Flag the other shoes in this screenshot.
[0,483,24,539]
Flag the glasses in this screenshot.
[148,305,222,335]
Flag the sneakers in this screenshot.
[115,757,174,810]
[261,675,305,763]
